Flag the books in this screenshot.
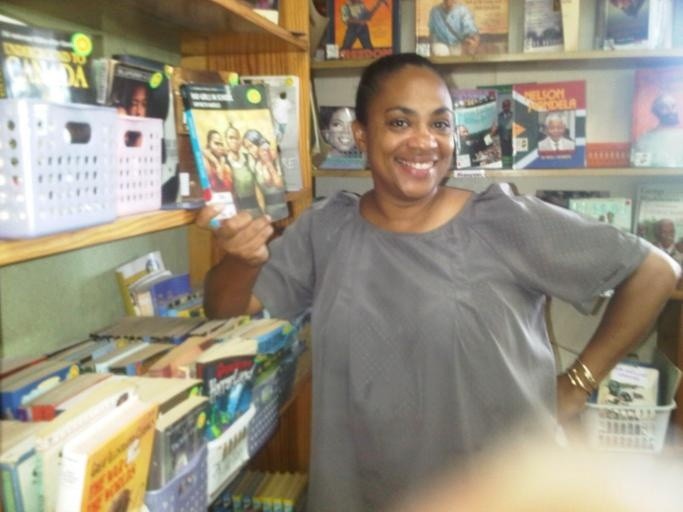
[317,2,682,260]
[2,16,302,231]
[597,350,682,420]
[1,249,309,512]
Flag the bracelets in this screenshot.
[566,359,598,398]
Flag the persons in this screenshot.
[197,54,682,512]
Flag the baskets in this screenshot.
[583,398,677,453]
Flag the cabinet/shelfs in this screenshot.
[310,51,683,301]
[0,0,312,512]
